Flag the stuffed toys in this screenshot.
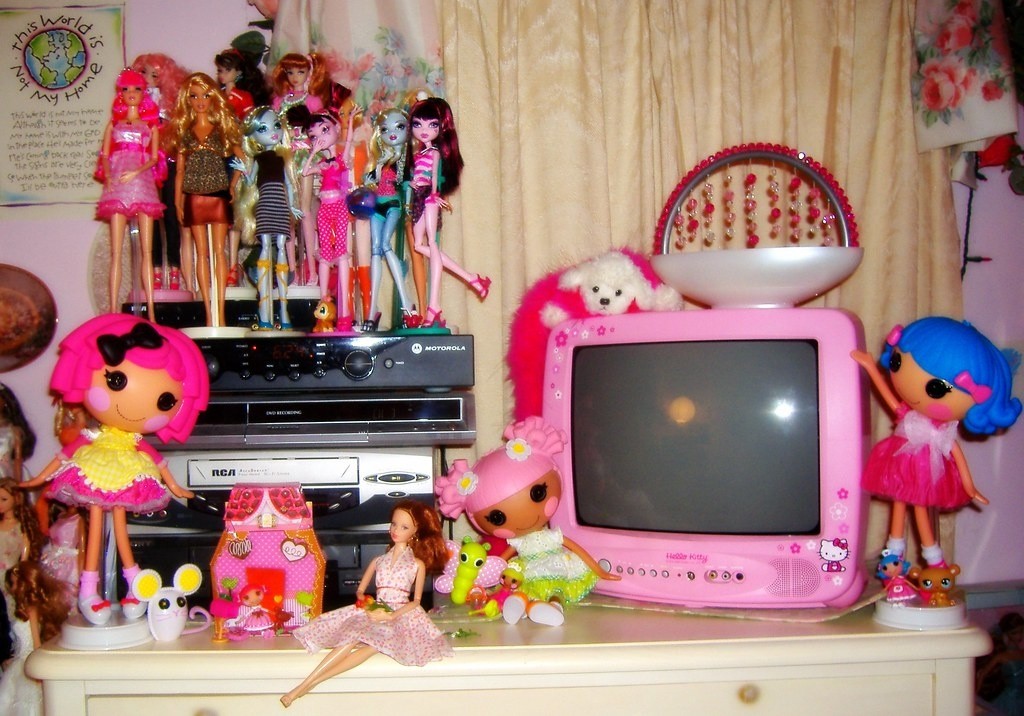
[502,245,685,424]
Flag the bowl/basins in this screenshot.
[650,246,864,309]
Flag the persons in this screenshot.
[235,582,275,636]
[93,48,491,331]
[0,384,88,716]
[279,498,453,708]
[850,316,1022,586]
[876,554,915,605]
[436,415,622,628]
[19,313,210,623]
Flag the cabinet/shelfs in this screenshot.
[26,597,992,716]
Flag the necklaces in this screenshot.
[420,147,436,153]
[284,94,307,104]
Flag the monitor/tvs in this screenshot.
[541,307,873,607]
